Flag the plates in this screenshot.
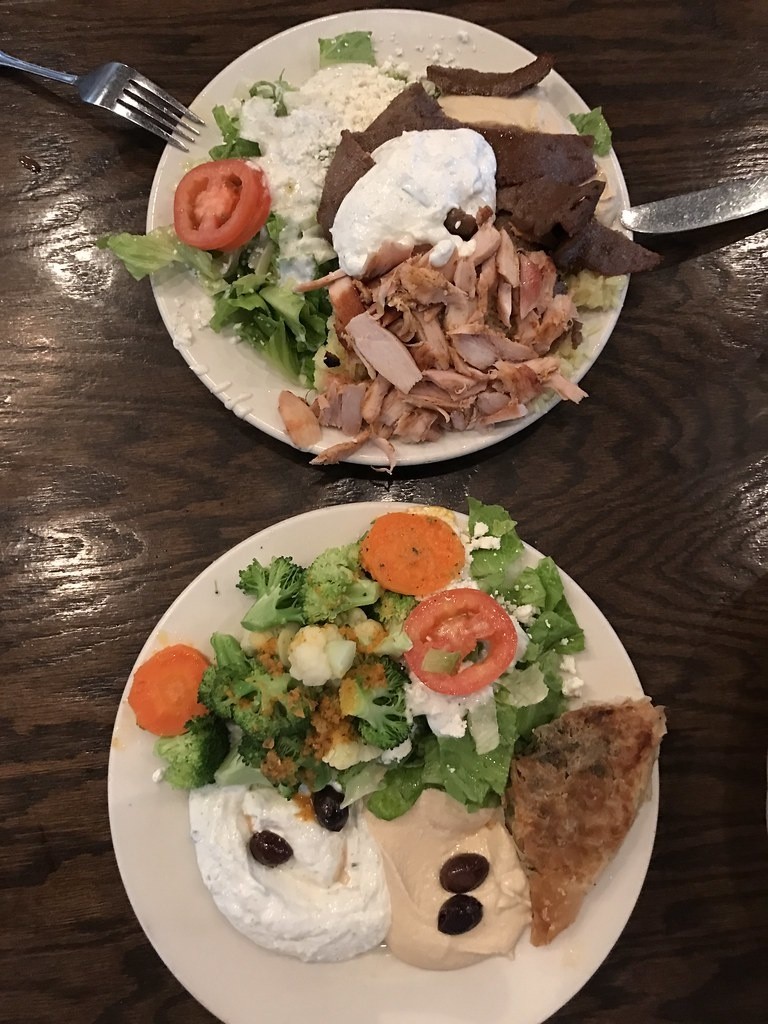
[146,8,636,469]
[105,501,661,1024]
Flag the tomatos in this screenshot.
[221,160,271,252]
[402,588,519,696]
[173,160,256,250]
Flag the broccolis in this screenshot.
[151,531,417,798]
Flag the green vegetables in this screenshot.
[345,499,586,820]
[108,28,383,387]
[569,107,614,155]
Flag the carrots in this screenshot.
[364,512,468,596]
[128,644,212,736]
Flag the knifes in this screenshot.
[619,172,768,234]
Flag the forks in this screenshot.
[0,50,206,153]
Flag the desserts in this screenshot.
[507,697,669,947]
